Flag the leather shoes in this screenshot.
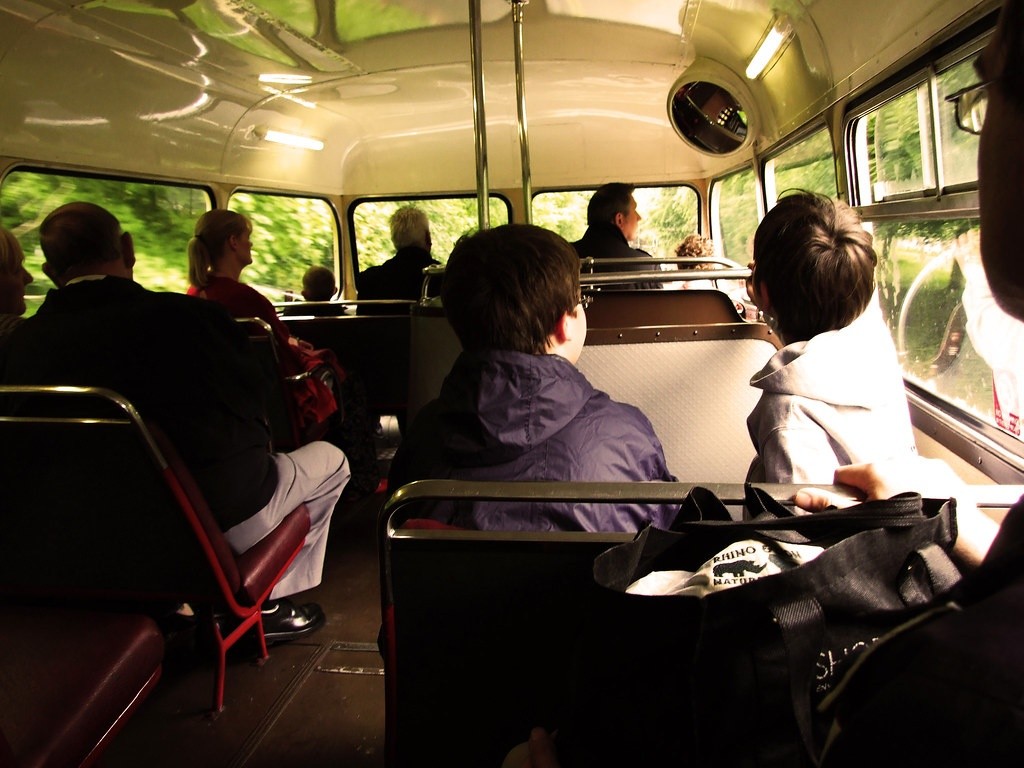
[221,602,327,643]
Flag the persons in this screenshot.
[502,0,1024,768]
[0,200,352,641]
[379,223,683,660]
[280,180,745,315]
[734,187,919,522]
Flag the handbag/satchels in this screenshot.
[592,484,963,768]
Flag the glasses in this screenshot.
[943,69,1007,134]
[577,294,595,308]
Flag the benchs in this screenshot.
[0,259,1024,768]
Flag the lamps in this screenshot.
[744,14,795,80]
